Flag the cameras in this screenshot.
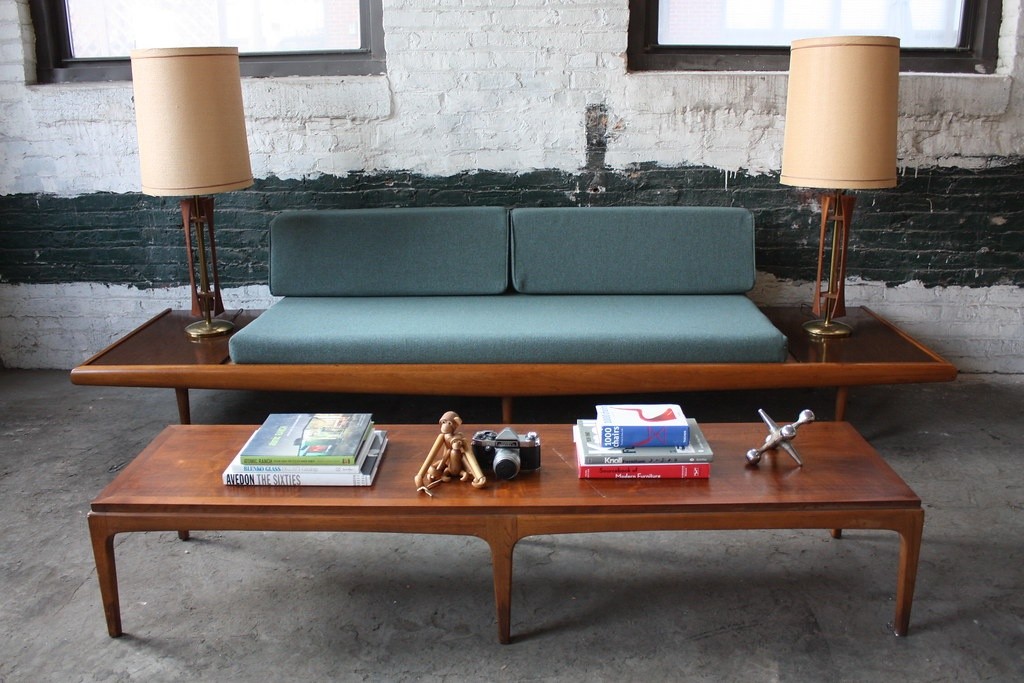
[470,427,541,479]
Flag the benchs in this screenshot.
[69,205,959,429]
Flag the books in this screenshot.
[571,404,715,479]
[222,413,389,486]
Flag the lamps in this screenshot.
[129,46,252,338]
[780,34,901,341]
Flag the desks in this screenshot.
[87,425,926,648]
